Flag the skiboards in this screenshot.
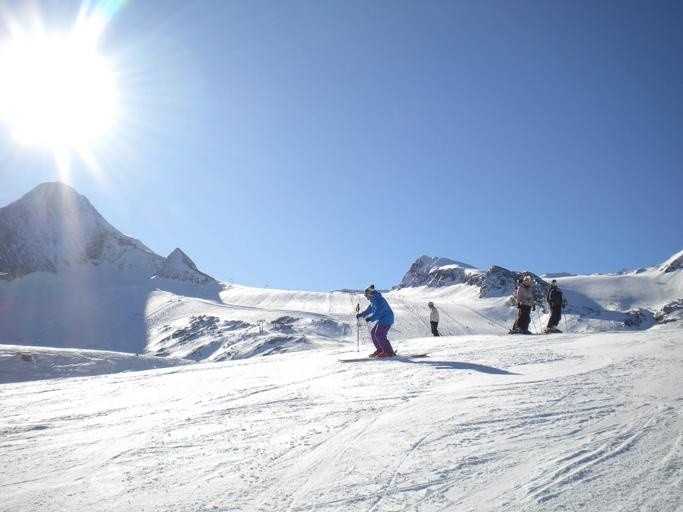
[337,353,432,362]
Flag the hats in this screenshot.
[365,284,377,294]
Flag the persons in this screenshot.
[543,279,563,333]
[508,315,531,335]
[356,284,397,357]
[515,276,536,332]
[427,302,440,336]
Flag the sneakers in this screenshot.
[515,328,532,334]
[545,328,562,333]
[369,350,394,357]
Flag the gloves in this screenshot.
[532,305,536,311]
[517,303,521,308]
[356,313,362,319]
[366,318,370,322]
